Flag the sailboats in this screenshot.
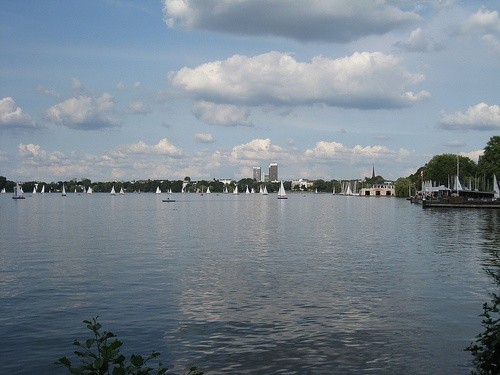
[232,186,240,195]
[110,186,116,195]
[40,185,45,194]
[119,187,125,195]
[11,181,26,199]
[245,185,251,195]
[1,187,6,194]
[259,185,269,195]
[155,186,162,194]
[250,188,255,194]
[181,187,185,194]
[167,187,173,195]
[278,180,288,199]
[86,187,93,195]
[332,181,360,196]
[32,186,37,195]
[61,183,67,196]
[314,187,319,194]
[206,187,212,194]
